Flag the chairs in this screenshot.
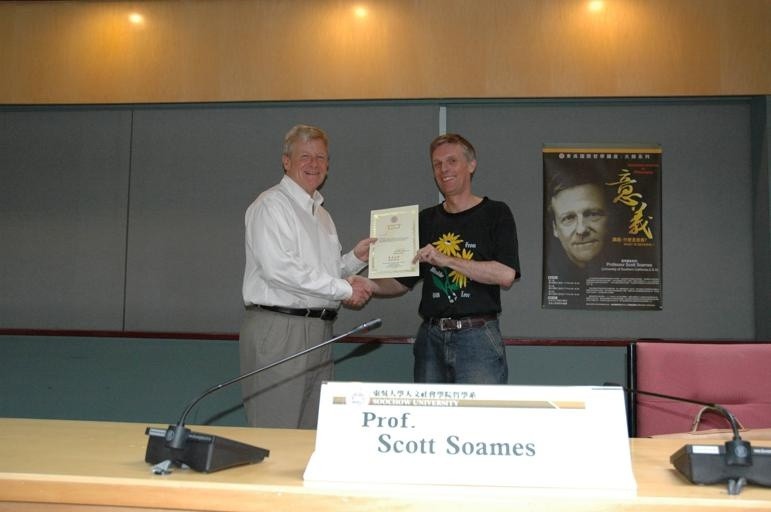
[627,337,771,438]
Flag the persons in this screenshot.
[345,133,523,385]
[238,125,380,430]
[548,166,648,292]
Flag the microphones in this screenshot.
[141,316,385,475]
[602,381,770,490]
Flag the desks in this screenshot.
[1,417,770,510]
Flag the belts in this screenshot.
[421,315,497,331]
[255,302,339,322]
